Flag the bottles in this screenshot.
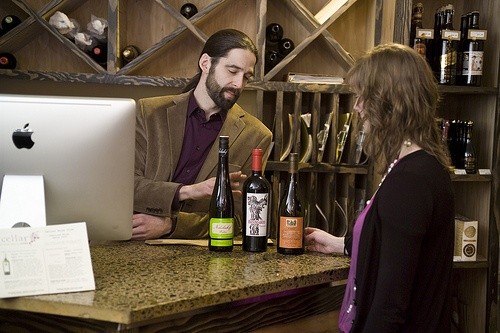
[435,119,476,174]
[409,2,484,87]
[242,149,272,253]
[208,136,234,253]
[50,12,109,53]
[181,2,198,19]
[265,51,282,73]
[123,45,139,63]
[277,152,305,255]
[0,51,17,69]
[0,15,21,36]
[92,47,107,68]
[266,23,283,46]
[276,38,295,56]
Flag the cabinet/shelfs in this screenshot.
[0,0,263,91]
[265,0,500,332]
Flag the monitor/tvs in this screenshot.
[0,94,136,244]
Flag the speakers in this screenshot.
[454,219,479,262]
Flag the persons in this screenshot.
[131,28,274,242]
[304,42,460,333]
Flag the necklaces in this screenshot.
[366,139,413,205]
